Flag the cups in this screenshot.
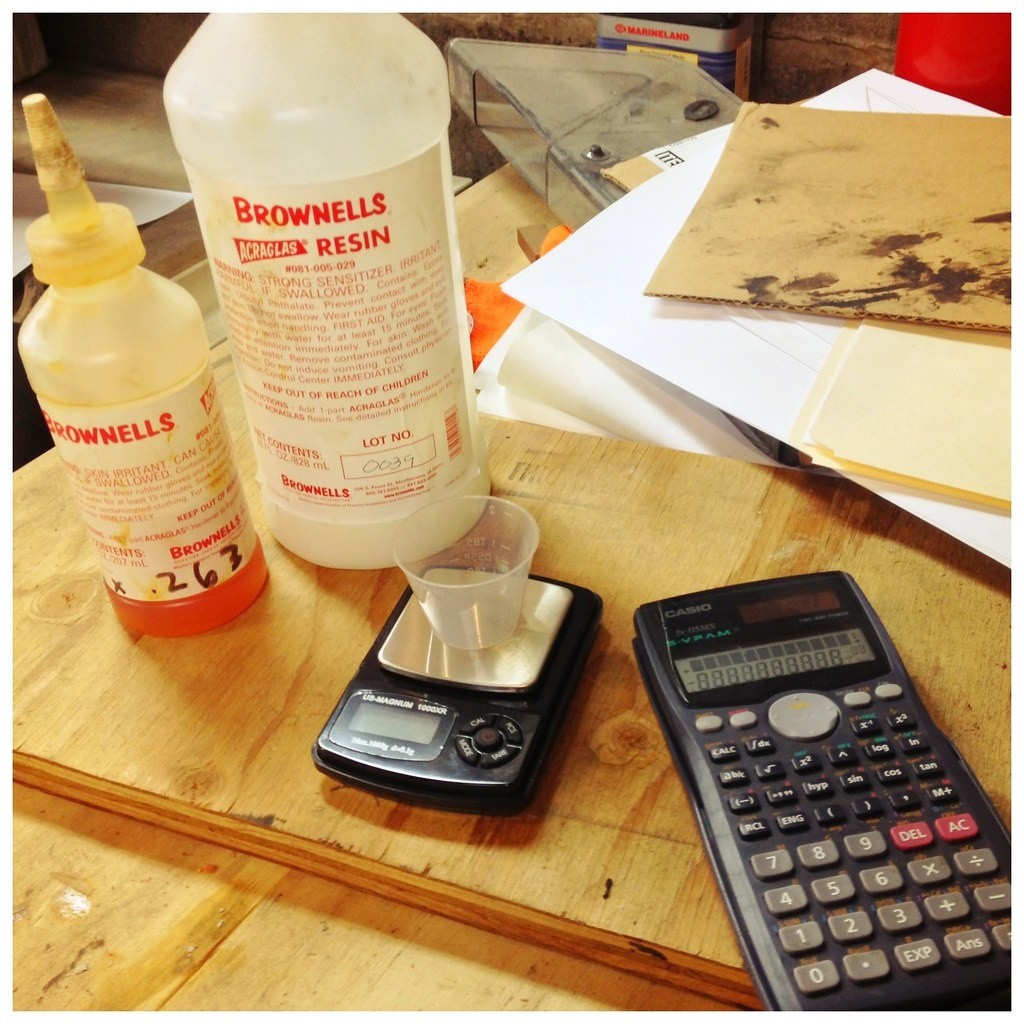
[393,494,539,649]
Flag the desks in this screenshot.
[12,165,1012,1011]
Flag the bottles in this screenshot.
[162,13,495,572]
[17,97,268,640]
